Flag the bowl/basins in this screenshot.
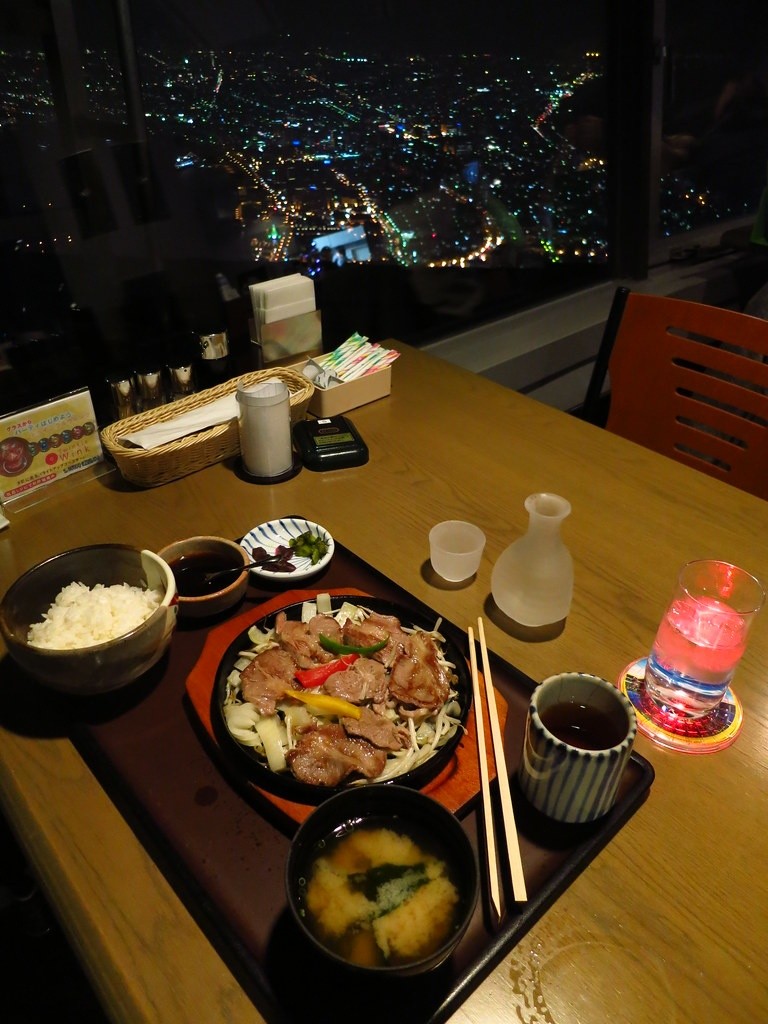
[157,535,250,616]
[0,543,181,697]
[285,783,481,979]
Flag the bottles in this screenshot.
[490,492,574,628]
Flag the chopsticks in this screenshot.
[467,616,530,928]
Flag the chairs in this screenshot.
[581,286,768,503]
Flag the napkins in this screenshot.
[247,273,316,326]
[119,374,294,451]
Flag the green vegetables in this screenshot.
[347,862,433,920]
[289,530,328,564]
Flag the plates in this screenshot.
[217,594,472,799]
[240,518,335,579]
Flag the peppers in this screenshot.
[282,690,361,720]
[294,652,360,688]
[317,633,391,653]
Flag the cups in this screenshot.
[520,671,638,825]
[235,381,293,478]
[429,520,486,582]
[644,559,765,719]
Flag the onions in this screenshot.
[225,592,467,784]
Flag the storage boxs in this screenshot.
[286,350,392,419]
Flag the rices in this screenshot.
[27,578,164,649]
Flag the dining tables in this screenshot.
[0,337,768,1024]
